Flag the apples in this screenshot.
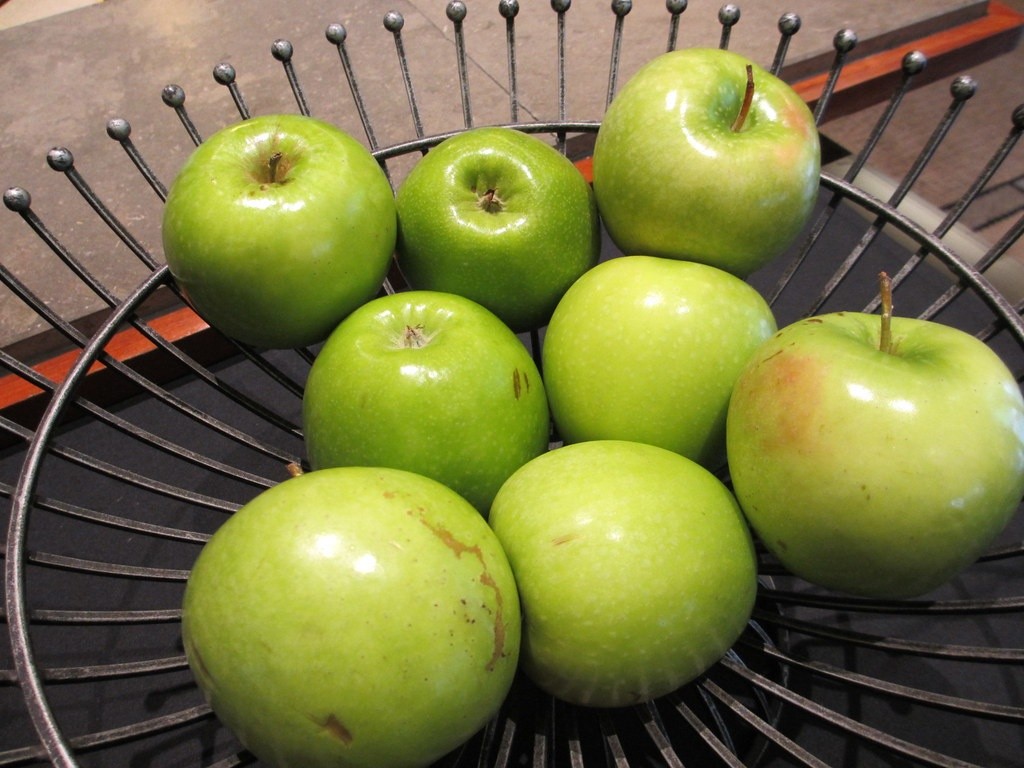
[391,126,601,333]
[161,115,398,348]
[587,45,820,280]
[541,255,778,475]
[301,291,552,523]
[725,272,1024,598]
[490,441,756,707]
[181,461,523,768]
